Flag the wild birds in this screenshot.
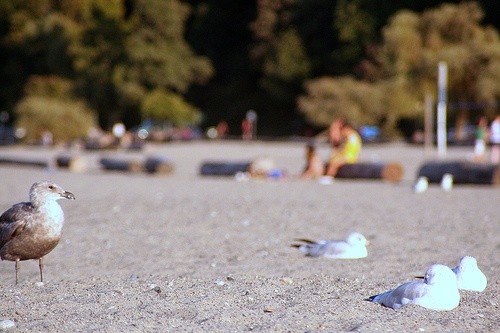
[364,263,461,312]
[415,255,488,295]
[1,180,76,286]
[289,231,370,259]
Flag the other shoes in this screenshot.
[318,175,335,185]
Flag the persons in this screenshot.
[0,112,255,151]
[301,113,500,184]
